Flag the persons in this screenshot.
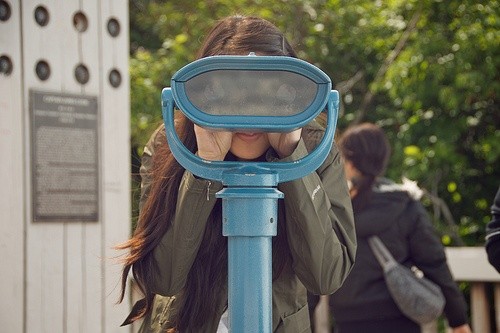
[485,196,500,274]
[117,15,357,333]
[305,123,471,332]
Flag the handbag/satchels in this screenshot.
[367,234,445,323]
